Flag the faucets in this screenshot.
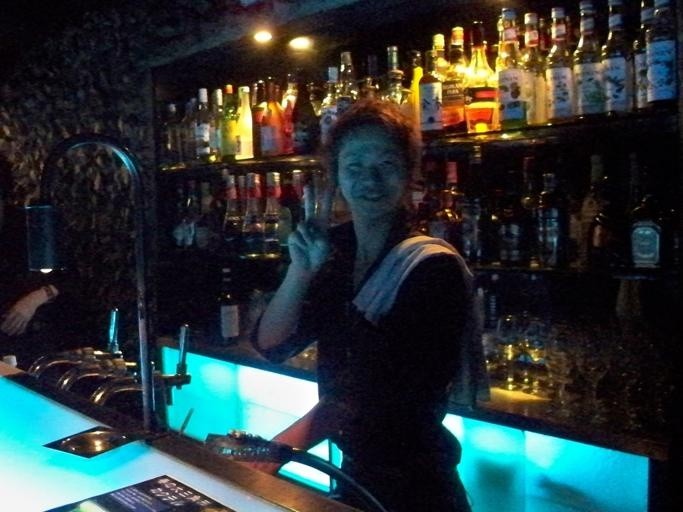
[25,308,191,407]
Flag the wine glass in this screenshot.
[478,315,647,436]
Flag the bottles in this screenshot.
[157,1,676,165]
[214,260,243,345]
[166,154,669,271]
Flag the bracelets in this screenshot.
[42,283,56,304]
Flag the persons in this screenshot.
[239,95,471,512]
[1,143,94,394]
[159,167,259,333]
